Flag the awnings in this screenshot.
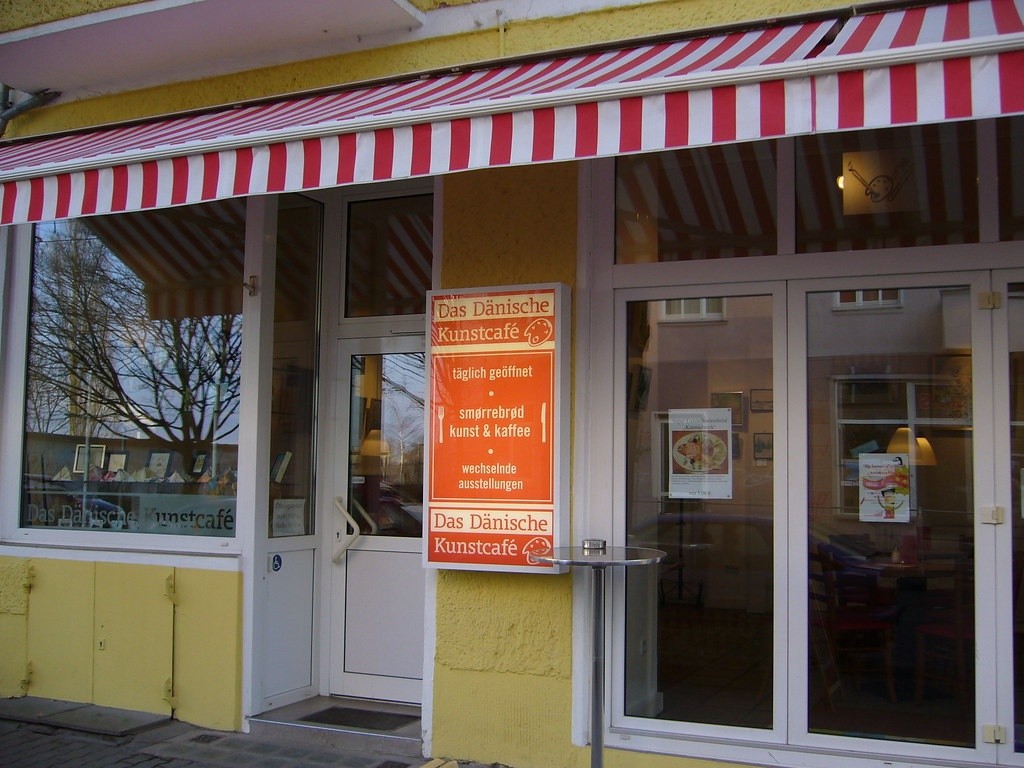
[2,1,1024,223]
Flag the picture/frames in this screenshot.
[750,389,773,411]
[628,364,653,413]
[72,444,107,474]
[733,433,740,457]
[927,353,973,438]
[145,450,173,479]
[107,451,129,474]
[188,451,208,476]
[754,433,773,459]
[270,451,292,483]
[710,391,744,427]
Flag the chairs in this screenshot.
[913,533,975,663]
[914,554,1021,704]
[828,533,902,645]
[816,542,898,705]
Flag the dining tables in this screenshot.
[842,555,968,692]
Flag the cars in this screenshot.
[20,473,127,531]
[349,476,422,536]
[627,512,929,669]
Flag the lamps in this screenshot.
[360,420,391,457]
[884,427,937,465]
